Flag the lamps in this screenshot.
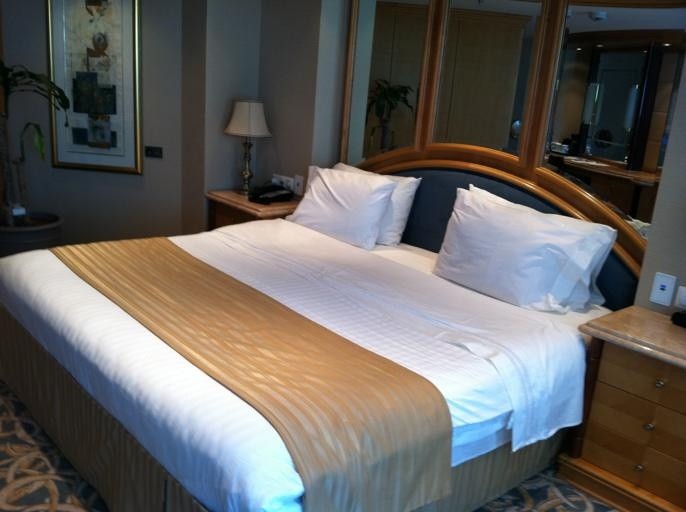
[224,100,272,195]
[583,82,599,125]
[622,81,641,132]
[593,83,604,125]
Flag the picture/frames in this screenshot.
[45,0,143,176]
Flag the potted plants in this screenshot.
[0,60,70,259]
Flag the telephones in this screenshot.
[248,185,293,205]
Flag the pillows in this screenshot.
[468,184,618,311]
[429,187,614,312]
[332,163,423,247]
[284,165,395,252]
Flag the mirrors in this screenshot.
[574,40,647,170]
[340,1,434,165]
[525,2,685,245]
[419,2,548,169]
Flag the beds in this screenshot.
[1,143,649,511]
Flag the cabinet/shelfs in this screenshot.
[361,3,533,159]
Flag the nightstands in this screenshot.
[204,189,302,232]
[556,306,686,512]
